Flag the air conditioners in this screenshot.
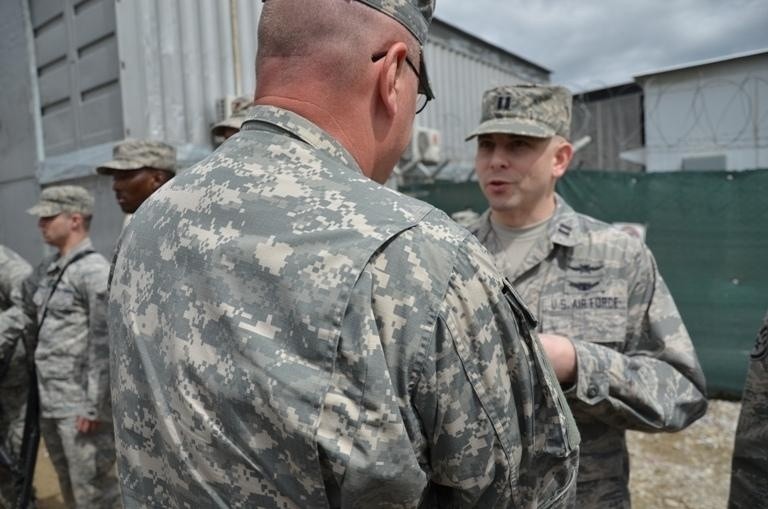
[400,125,441,163]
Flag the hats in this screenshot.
[464,80,573,144]
[25,184,95,218]
[210,94,255,133]
[95,138,178,176]
[360,0,437,99]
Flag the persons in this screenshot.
[0,245,34,508]
[464,83,707,509]
[725,324,768,508]
[23,185,122,508]
[96,139,177,214]
[105,0,581,509]
[210,97,253,138]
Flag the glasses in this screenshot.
[372,49,429,117]
